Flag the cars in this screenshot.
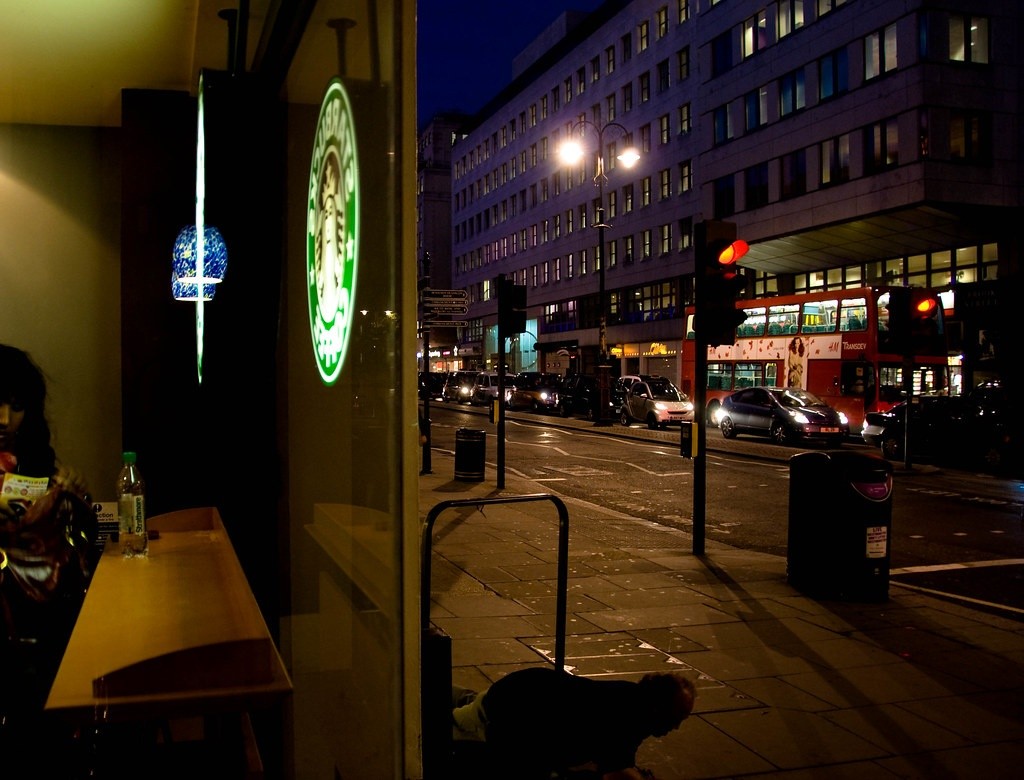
[716,384,850,448]
[967,379,1024,425]
[618,380,695,429]
[858,393,966,446]
[416,365,633,424]
[616,373,664,405]
[880,408,1024,475]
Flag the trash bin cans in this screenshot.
[785,449,895,606]
[454,427,487,482]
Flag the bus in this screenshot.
[680,285,951,444]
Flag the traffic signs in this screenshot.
[422,289,469,328]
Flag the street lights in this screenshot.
[560,120,640,362]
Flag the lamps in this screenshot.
[172,271,216,302]
[172,224,228,285]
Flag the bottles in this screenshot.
[117,452,148,554]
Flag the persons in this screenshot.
[788,337,804,392]
[0,343,88,644]
[452,667,697,780]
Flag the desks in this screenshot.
[44,507,295,780]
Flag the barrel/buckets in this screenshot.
[454,427,487,482]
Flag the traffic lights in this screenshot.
[698,215,748,348]
[891,284,944,356]
[499,275,528,338]
